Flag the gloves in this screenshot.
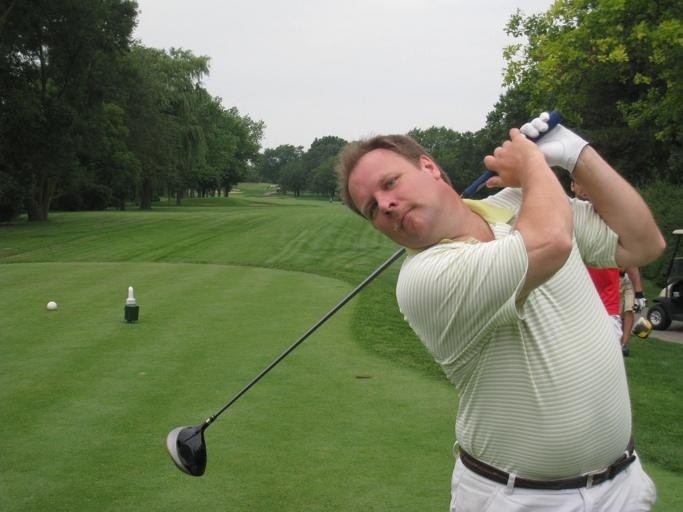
[632,297,648,314]
[519,111,590,174]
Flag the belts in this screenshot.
[459,437,636,490]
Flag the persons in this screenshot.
[616,264,646,357]
[335,110,669,512]
[567,175,647,313]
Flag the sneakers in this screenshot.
[622,346,630,357]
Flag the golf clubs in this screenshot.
[166,110,560,478]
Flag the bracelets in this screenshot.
[635,291,644,300]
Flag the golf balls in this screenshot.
[46,302,57,311]
